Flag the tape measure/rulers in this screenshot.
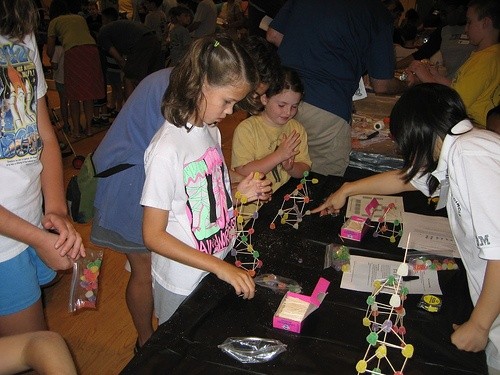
[416,294,442,312]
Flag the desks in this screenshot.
[118,171,490,375]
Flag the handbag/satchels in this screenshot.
[65,144,137,224]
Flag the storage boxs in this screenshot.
[341,198,379,241]
[273,276,329,333]
[352,79,404,155]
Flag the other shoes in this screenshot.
[92,117,109,127]
[87,130,94,136]
[107,105,116,114]
[109,111,119,118]
[57,123,71,135]
[73,136,81,140]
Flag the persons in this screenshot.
[0,331,79,375]
[40,0,500,375]
[0,0,88,340]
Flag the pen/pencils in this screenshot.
[374,276,419,282]
